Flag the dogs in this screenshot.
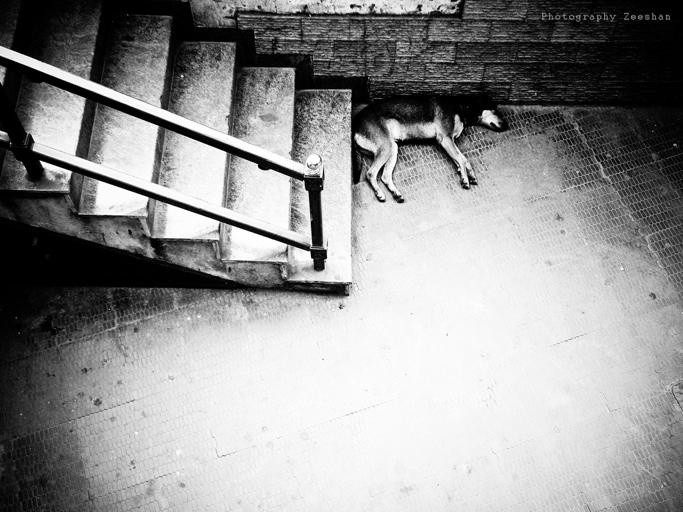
[351,91,507,204]
